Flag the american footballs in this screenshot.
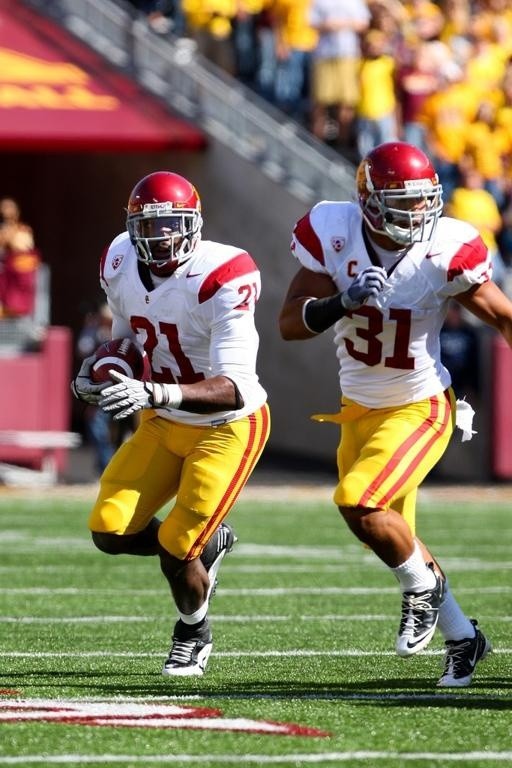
[90,337,143,395]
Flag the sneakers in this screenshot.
[199,522,239,604]
[161,618,214,679]
[394,560,450,659]
[435,617,491,689]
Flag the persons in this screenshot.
[0,191,41,320]
[271,137,510,693]
[68,170,271,680]
[134,1,511,294]
[79,303,118,477]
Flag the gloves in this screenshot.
[341,265,389,311]
[98,369,172,421]
[70,354,112,406]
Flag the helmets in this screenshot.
[125,170,202,245]
[356,139,441,230]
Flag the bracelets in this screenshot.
[302,290,348,335]
